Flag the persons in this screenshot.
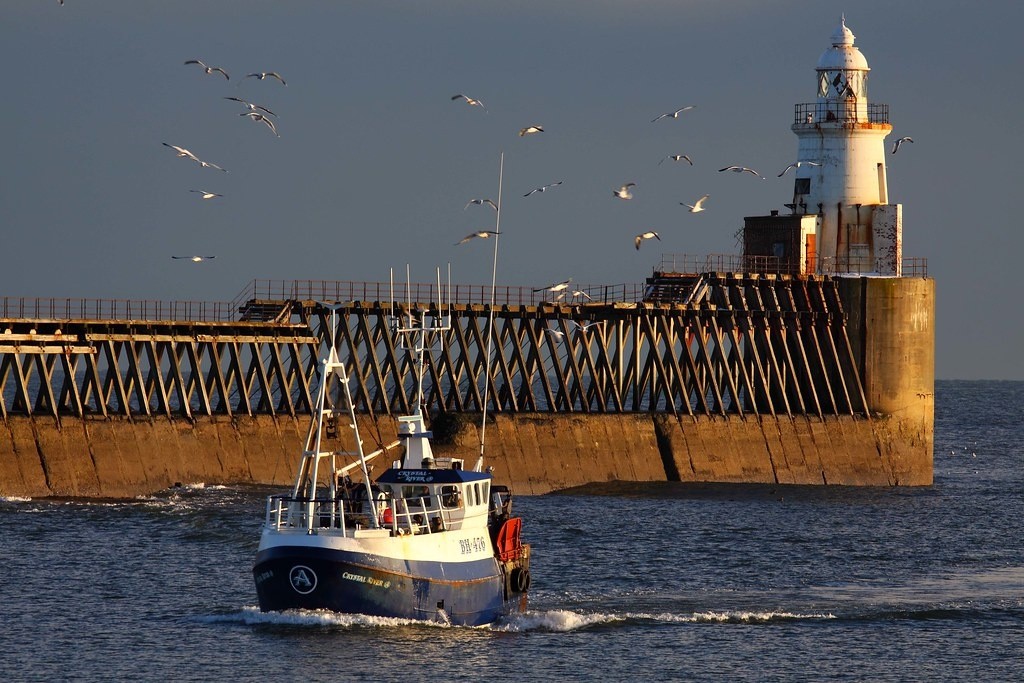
[490,512,510,555]
[416,487,430,506]
[808,112,813,123]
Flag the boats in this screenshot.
[252,151,531,627]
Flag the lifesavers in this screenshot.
[511,567,526,593]
[524,570,532,595]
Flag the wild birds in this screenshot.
[657,153,693,167]
[633,230,661,250]
[717,166,765,180]
[891,137,914,155]
[454,230,503,247]
[531,279,606,343]
[777,159,821,177]
[648,104,697,124]
[518,125,544,138]
[463,198,499,211]
[613,182,636,200]
[451,94,489,112]
[524,181,564,196]
[162,142,230,174]
[188,189,223,200]
[679,193,709,213]
[171,255,216,262]
[184,59,288,138]
